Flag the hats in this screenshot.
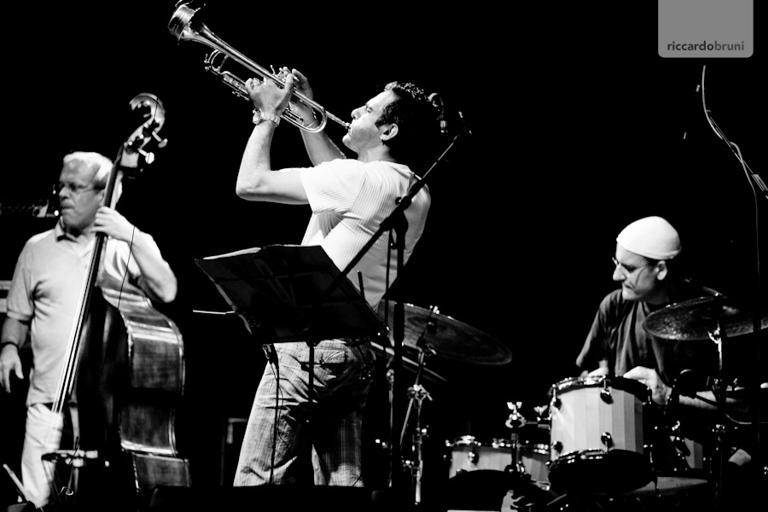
[615,214,683,262]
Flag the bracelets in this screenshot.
[306,118,319,128]
[666,387,680,406]
[0,341,20,353]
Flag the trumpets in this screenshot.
[168,4,350,133]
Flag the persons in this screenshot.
[572,216,753,413]
[231,67,436,487]
[0,150,176,512]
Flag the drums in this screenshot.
[627,476,708,511]
[442,436,551,487]
[547,375,649,498]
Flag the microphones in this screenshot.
[428,92,446,135]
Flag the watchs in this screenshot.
[252,107,280,126]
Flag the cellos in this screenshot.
[40,93,192,512]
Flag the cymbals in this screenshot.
[643,296,768,341]
[377,298,512,366]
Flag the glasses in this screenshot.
[52,181,95,195]
